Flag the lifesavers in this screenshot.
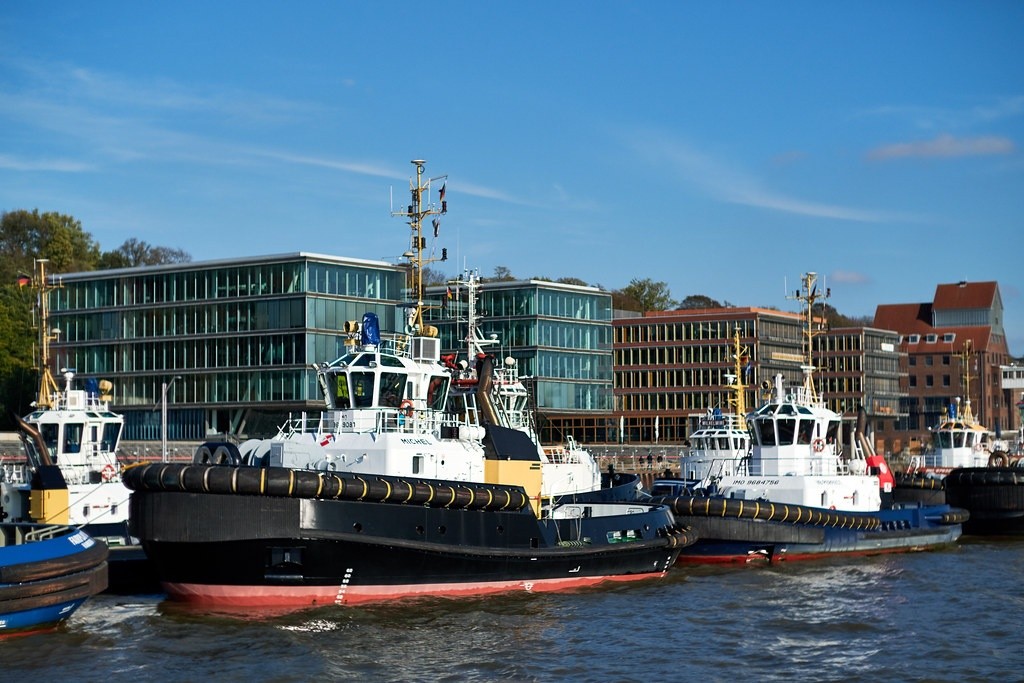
[813,438,824,451]
[990,452,1008,469]
[102,464,115,480]
[400,400,413,416]
[975,443,982,452]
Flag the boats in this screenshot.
[0,260,150,595]
[642,268,1024,566]
[0,409,109,642]
[118,154,701,607]
